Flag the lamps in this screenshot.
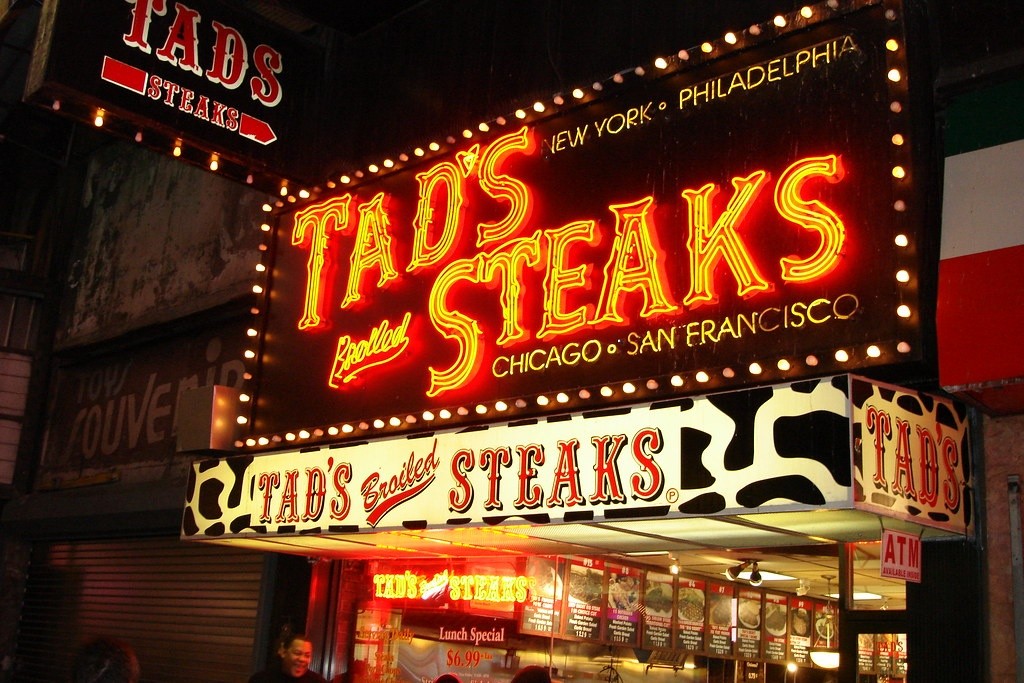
[670,561,682,576]
[805,574,839,669]
[724,562,753,583]
[749,561,763,587]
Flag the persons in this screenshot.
[434,674,461,683]
[248,633,326,683]
[511,665,552,683]
[70,636,139,683]
